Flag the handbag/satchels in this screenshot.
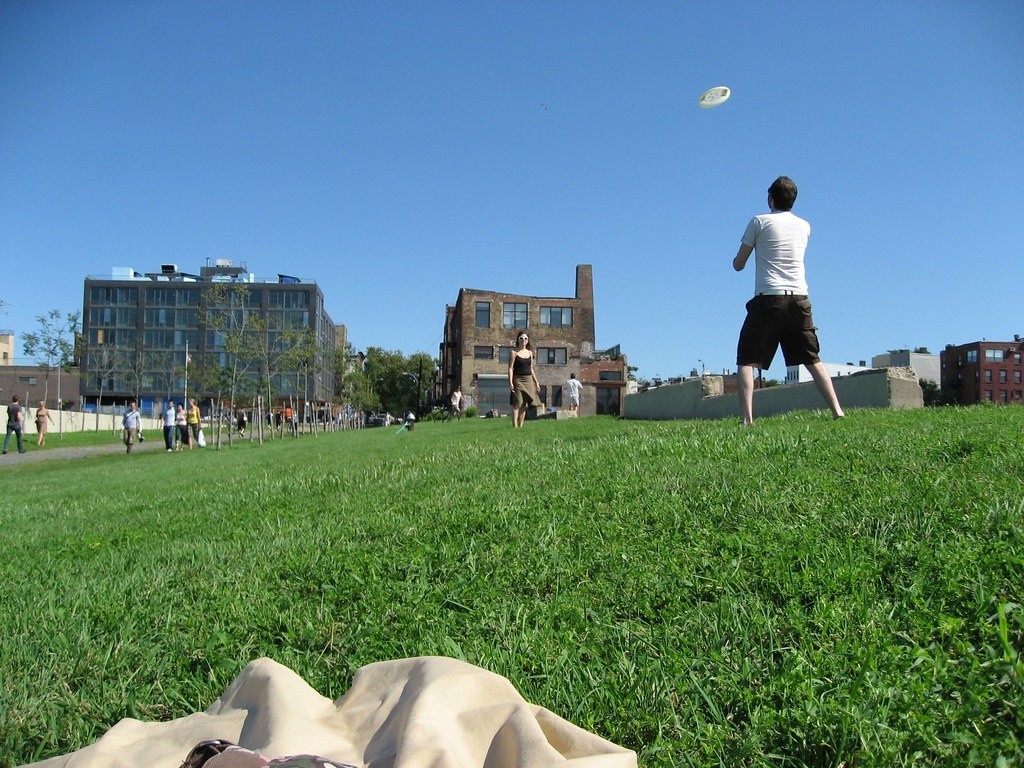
[197,428,206,448]
[137,432,145,443]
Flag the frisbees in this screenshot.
[699,86,731,109]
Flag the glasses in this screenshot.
[517,337,528,340]
[177,739,236,768]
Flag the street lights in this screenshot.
[698,359,705,374]
[412,353,422,422]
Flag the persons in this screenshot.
[733,174,845,426]
[2,395,26,454]
[160,400,176,452]
[34,400,55,447]
[187,397,203,450]
[265,407,274,434]
[563,373,584,412]
[119,401,145,454]
[383,411,391,427]
[507,330,541,428]
[236,405,248,438]
[276,410,283,432]
[174,403,188,451]
[292,409,299,434]
[447,385,466,423]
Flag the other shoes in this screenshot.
[3,451,7,454]
[168,448,172,453]
[19,450,25,453]
